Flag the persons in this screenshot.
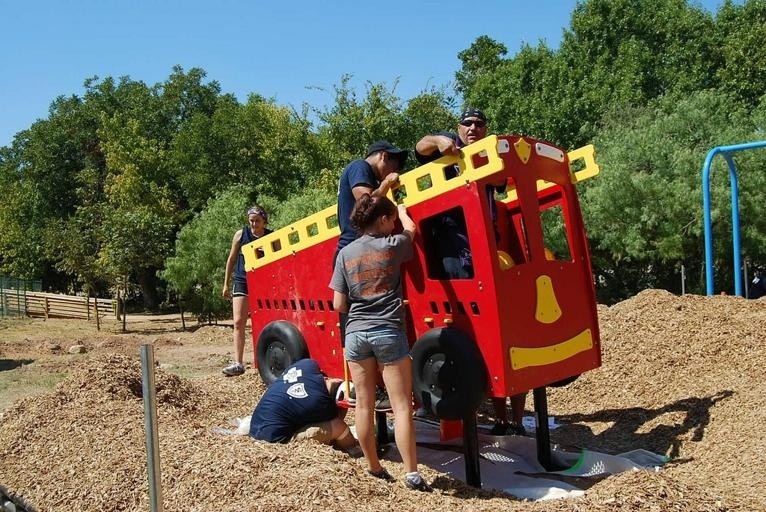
[329,194,436,495]
[413,106,513,284]
[250,358,357,450]
[331,139,408,351]
[221,204,275,379]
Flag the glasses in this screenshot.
[461,119,487,128]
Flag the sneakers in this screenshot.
[367,467,397,484]
[406,476,435,494]
[222,362,245,376]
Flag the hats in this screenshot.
[461,109,487,122]
[367,140,409,160]
